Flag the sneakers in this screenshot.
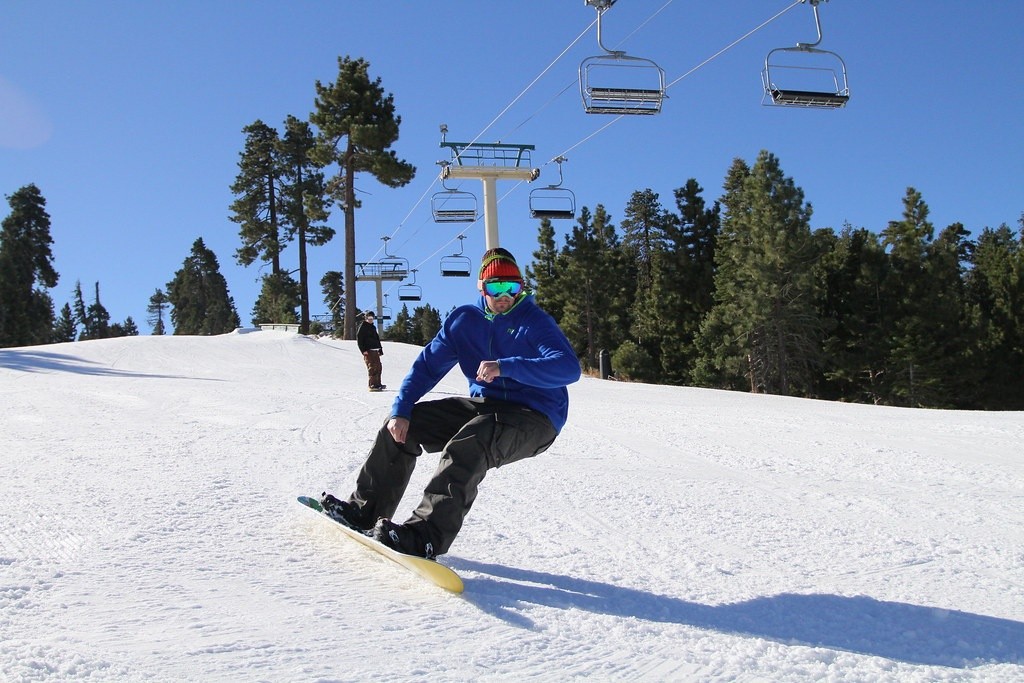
[320,492,376,536]
[374,517,435,560]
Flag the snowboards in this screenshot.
[368,385,386,392]
[295,496,465,595]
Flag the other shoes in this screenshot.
[377,385,386,388]
[369,387,382,392]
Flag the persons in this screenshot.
[356,310,387,389]
[320,246,582,562]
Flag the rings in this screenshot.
[482,374,486,378]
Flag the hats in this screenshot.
[479,248,521,299]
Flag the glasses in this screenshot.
[483,281,521,299]
